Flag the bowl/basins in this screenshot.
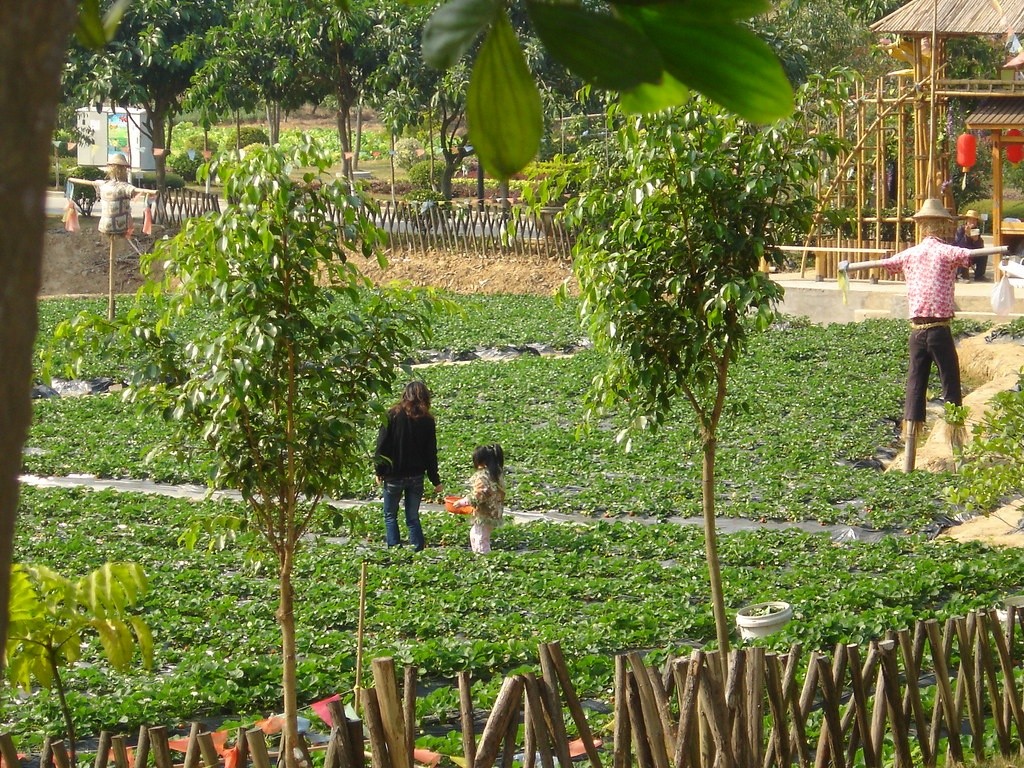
[970,229,980,236]
[445,497,472,514]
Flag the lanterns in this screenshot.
[1006,128,1024,170]
[957,132,977,190]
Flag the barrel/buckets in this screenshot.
[736,602,792,643]
[994,596,1024,623]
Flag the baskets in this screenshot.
[444,497,473,515]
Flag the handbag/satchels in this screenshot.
[375,454,394,478]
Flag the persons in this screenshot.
[69,154,161,236]
[451,443,505,556]
[374,380,443,550]
[838,200,1011,475]
[955,209,993,283]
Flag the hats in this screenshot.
[912,199,953,219]
[106,154,130,168]
[957,210,982,223]
